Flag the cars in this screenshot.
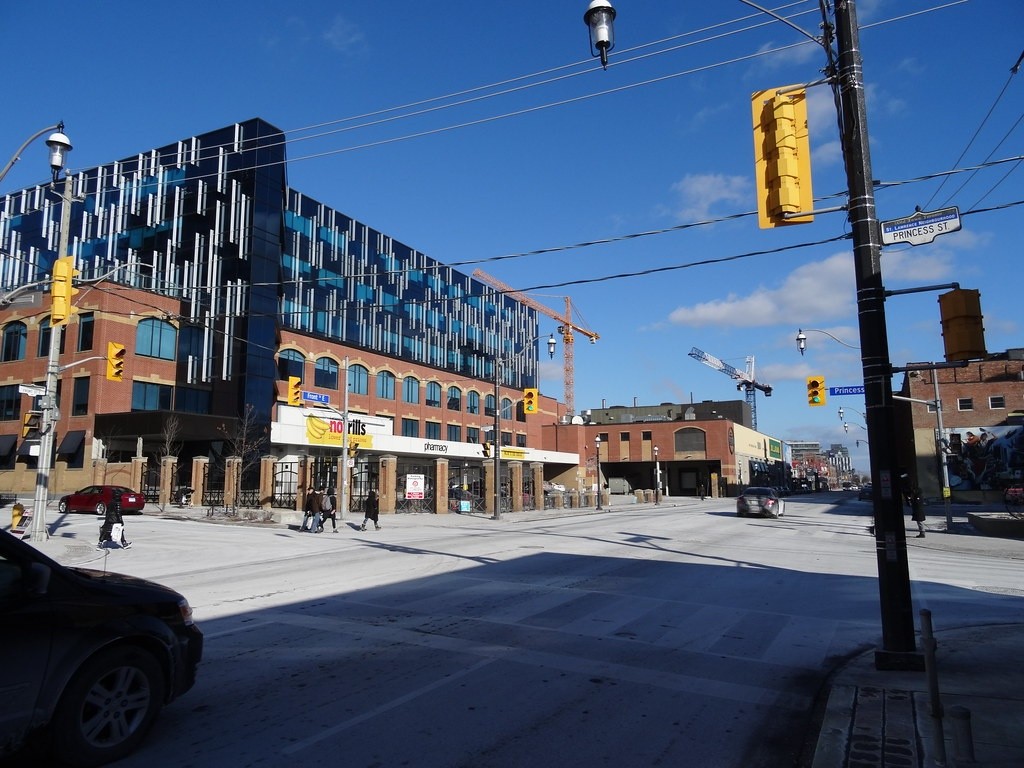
[859,483,872,500]
[737,487,785,518]
[543,481,565,494]
[59,486,145,515]
[0,528,203,768]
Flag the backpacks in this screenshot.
[321,495,334,512]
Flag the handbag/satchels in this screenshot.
[99,525,112,540]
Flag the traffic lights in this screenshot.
[288,376,301,406]
[350,442,359,458]
[524,389,538,414]
[482,442,490,457]
[807,375,826,406]
[49,256,79,327]
[107,341,126,382]
[22,412,41,439]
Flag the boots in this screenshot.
[362,524,367,531]
[374,523,382,530]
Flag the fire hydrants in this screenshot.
[12,503,24,529]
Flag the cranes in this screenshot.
[688,347,773,432]
[473,267,601,416]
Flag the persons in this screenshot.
[96,489,132,550]
[362,491,381,531]
[298,486,339,533]
[699,484,706,500]
[909,486,926,538]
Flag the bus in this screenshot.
[842,481,852,491]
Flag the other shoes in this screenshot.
[299,527,324,533]
[916,533,925,538]
[333,528,339,533]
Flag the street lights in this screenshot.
[491,333,556,520]
[738,460,742,494]
[653,445,660,505]
[595,435,603,510]
[585,0,924,671]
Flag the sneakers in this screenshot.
[97,547,107,552]
[123,542,133,550]
